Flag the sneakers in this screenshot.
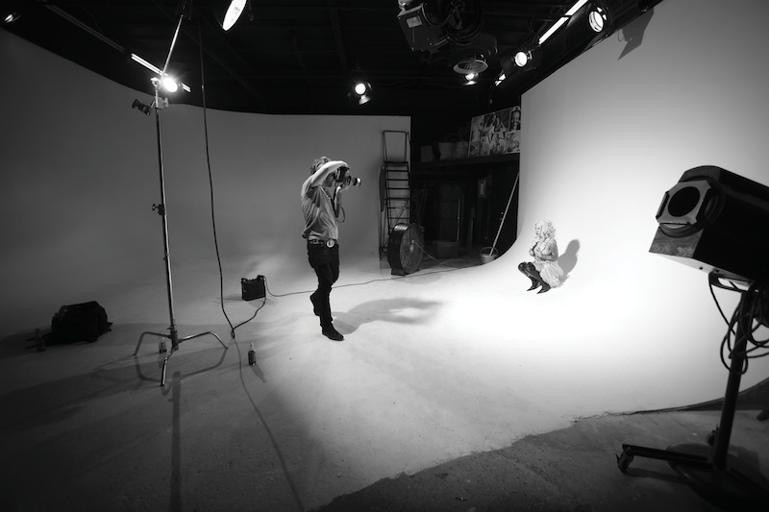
[310,294,320,316]
[322,325,344,340]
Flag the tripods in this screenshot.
[134,89,229,387]
[616,291,769,512]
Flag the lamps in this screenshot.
[515,48,535,69]
[216,0,249,32]
[346,79,374,106]
[491,0,588,87]
[585,0,617,34]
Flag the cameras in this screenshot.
[334,166,361,188]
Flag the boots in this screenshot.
[518,263,550,293]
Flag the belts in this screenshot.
[311,239,335,248]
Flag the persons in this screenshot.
[299,156,354,341]
[517,216,565,295]
[469,106,520,157]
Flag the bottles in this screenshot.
[158,328,167,354]
[247,343,256,366]
